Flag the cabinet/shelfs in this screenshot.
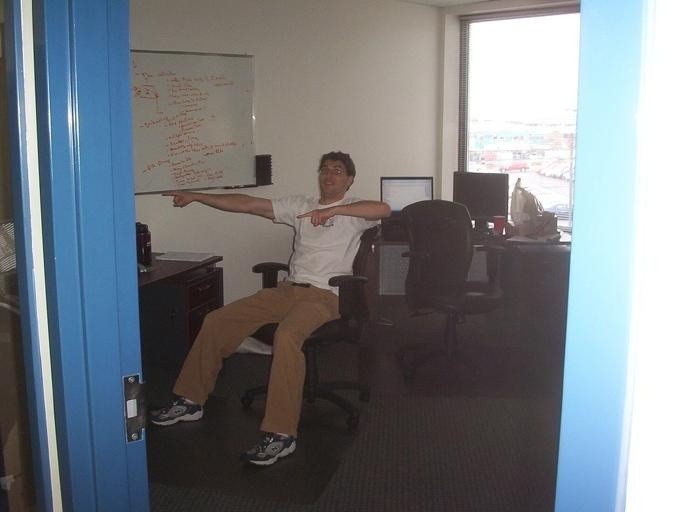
[150,267,222,368]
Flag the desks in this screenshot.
[139,247,225,373]
[361,218,571,352]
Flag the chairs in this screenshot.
[399,200,504,386]
[248,226,372,429]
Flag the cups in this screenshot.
[493,216,506,233]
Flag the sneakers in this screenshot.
[240,431,296,466]
[151,398,204,425]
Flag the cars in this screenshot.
[499,159,575,220]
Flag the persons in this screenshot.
[151,151,391,466]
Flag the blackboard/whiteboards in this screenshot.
[129,49,257,194]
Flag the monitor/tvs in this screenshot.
[453,171,509,227]
[380,176,434,213]
